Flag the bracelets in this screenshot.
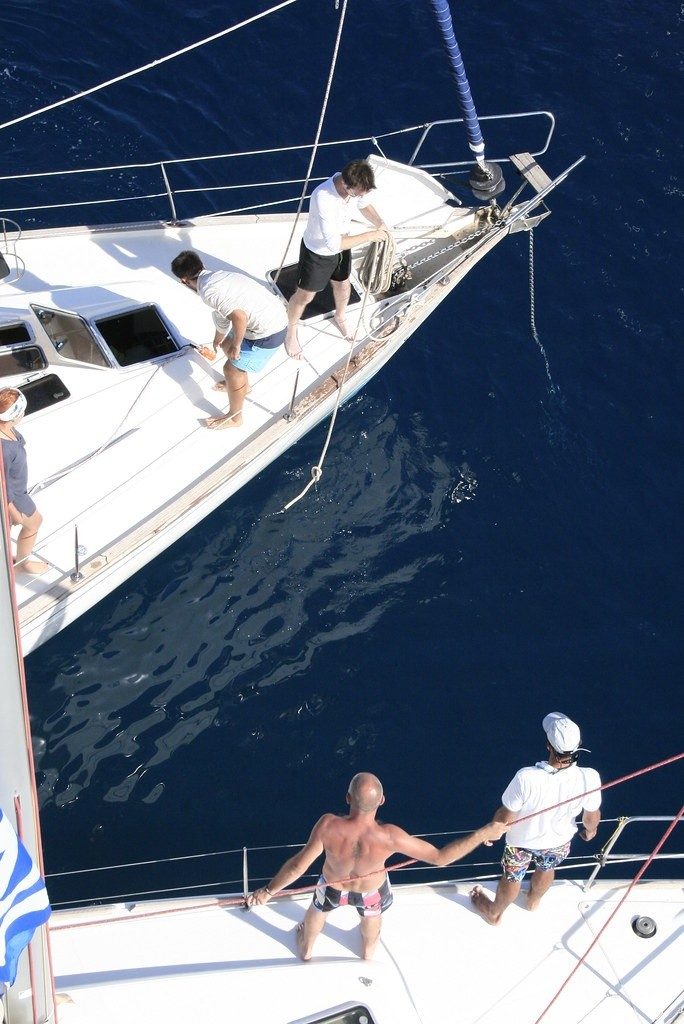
[265,885,275,896]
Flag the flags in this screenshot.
[0,812,51,1001]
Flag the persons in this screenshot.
[284,162,392,359]
[0,387,50,576]
[244,773,513,962]
[469,711,602,927]
[170,250,288,429]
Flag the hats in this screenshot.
[542,712,580,755]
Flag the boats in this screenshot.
[0,1,593,662]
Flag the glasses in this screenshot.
[349,188,363,199]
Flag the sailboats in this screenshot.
[0,428,683,1024]
